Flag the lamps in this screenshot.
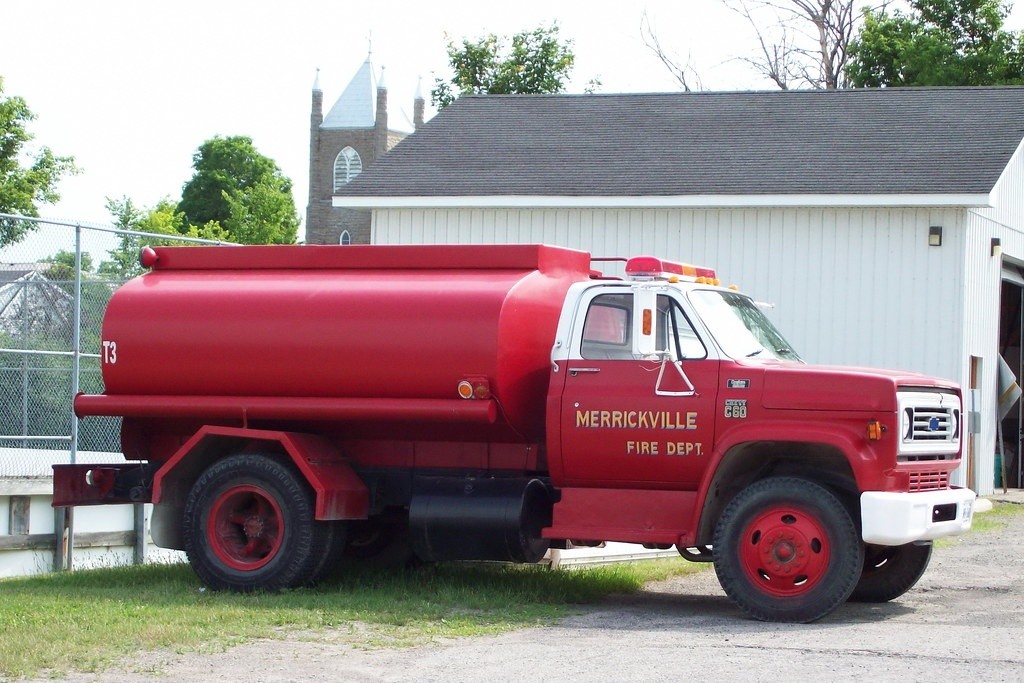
[928,225,943,247]
[991,237,1001,256]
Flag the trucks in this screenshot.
[50,242,978,630]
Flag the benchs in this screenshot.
[582,347,659,360]
